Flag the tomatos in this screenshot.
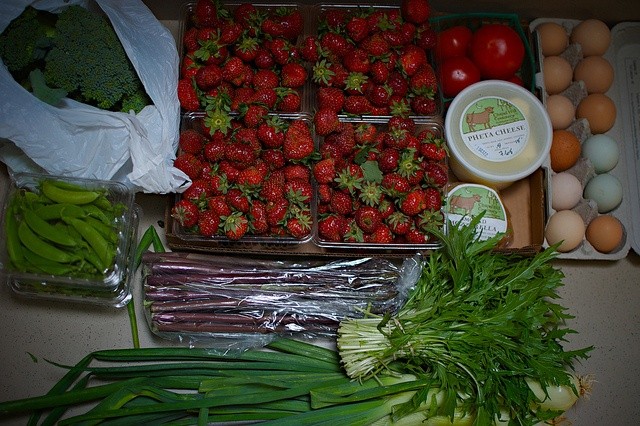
[468,23,525,78]
[438,57,483,96]
[435,23,472,64]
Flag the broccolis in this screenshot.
[0,0,151,115]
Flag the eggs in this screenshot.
[574,57,615,93]
[551,172,583,209]
[533,22,569,55]
[577,86,618,133]
[544,54,573,93]
[585,174,623,212]
[588,214,623,253]
[551,129,581,170]
[547,211,586,252]
[570,18,611,55]
[548,92,577,130]
[583,130,620,173]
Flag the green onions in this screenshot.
[1,335,601,425]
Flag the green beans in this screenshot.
[5,177,133,300]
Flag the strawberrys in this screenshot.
[170,1,450,244]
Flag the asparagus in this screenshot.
[140,252,399,342]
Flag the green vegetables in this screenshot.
[336,211,595,425]
